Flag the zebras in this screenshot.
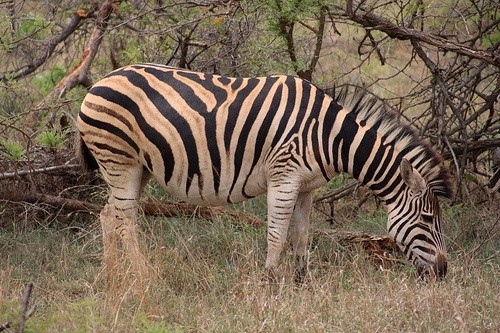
[74,62,455,286]
